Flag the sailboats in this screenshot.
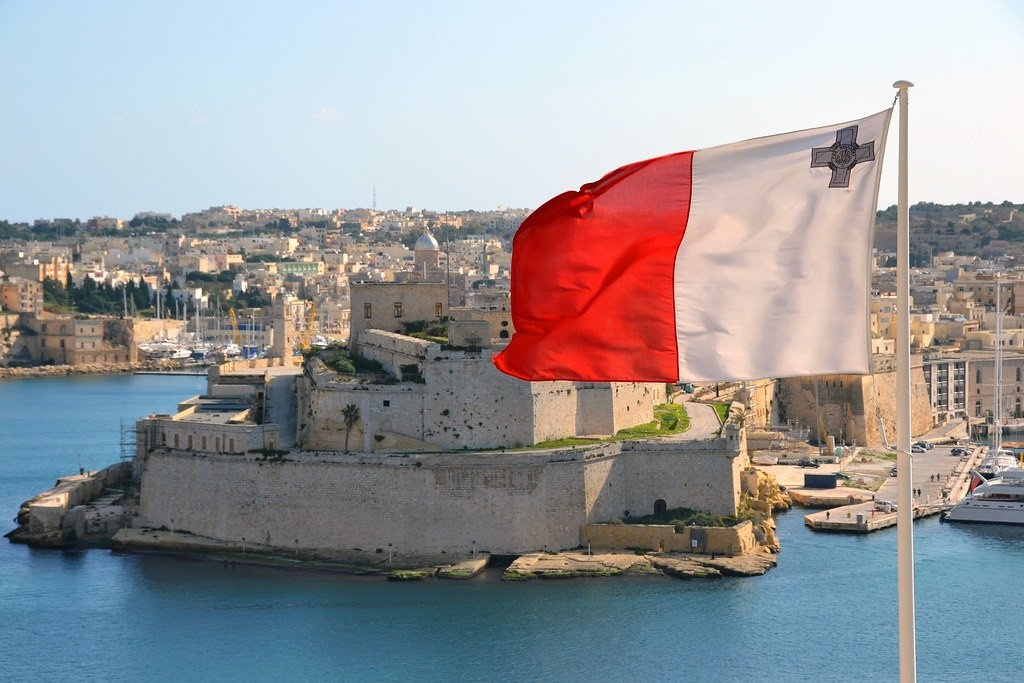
[938,272,1024,526]
[138,280,266,364]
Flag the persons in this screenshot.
[917,488,921,497]
[826,511,830,519]
[872,495,875,501]
[931,474,934,482]
[913,488,916,495]
[937,473,940,481]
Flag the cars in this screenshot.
[798,460,820,468]
[833,472,851,480]
[951,449,973,457]
[890,467,897,477]
[912,441,934,453]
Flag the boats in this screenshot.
[311,335,330,350]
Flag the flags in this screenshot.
[491,107,895,383]
[969,474,981,495]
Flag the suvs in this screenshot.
[873,499,897,512]
